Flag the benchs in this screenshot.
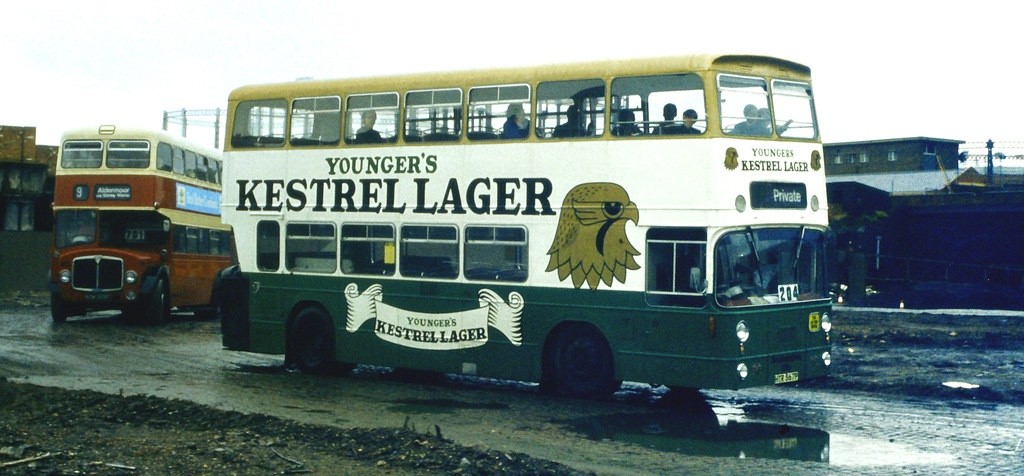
[258,252,336,271]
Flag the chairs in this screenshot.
[465,261,503,281]
[498,263,528,282]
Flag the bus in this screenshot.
[48,125,232,328]
[210,53,839,403]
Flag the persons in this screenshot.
[503,103,792,139]
[356,110,382,139]
[71,220,96,244]
